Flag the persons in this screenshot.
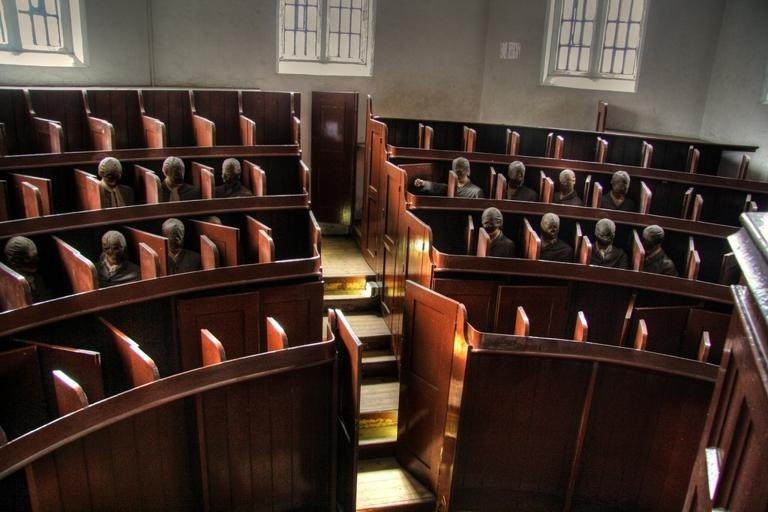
[92,231,140,287]
[215,158,252,198]
[160,156,201,202]
[553,169,581,206]
[601,170,636,211]
[590,218,628,269]
[506,161,538,202]
[162,218,201,275]
[481,207,514,258]
[414,157,484,198]
[539,213,573,263]
[98,157,133,208]
[4,236,40,302]
[642,225,679,277]
